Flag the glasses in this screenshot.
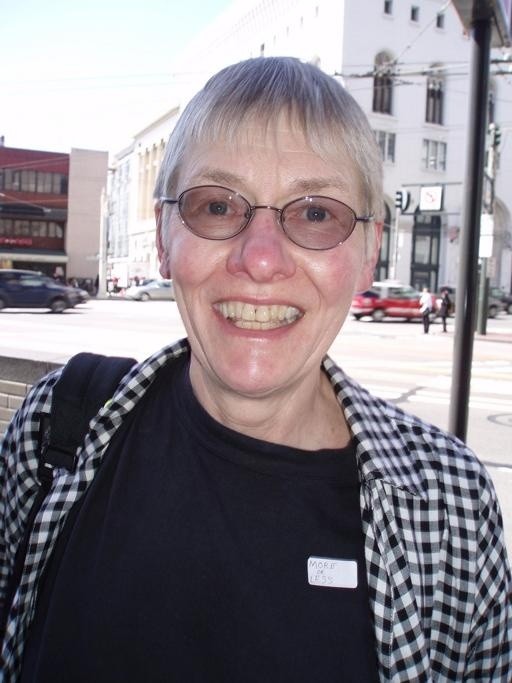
[160,185,375,250]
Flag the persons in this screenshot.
[0,56,512,683]
[436,286,452,331]
[418,286,432,333]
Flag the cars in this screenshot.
[352,279,443,323]
[432,287,510,319]
[0,268,92,312]
[127,278,177,302]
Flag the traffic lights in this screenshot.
[489,122,501,147]
[394,187,403,209]
